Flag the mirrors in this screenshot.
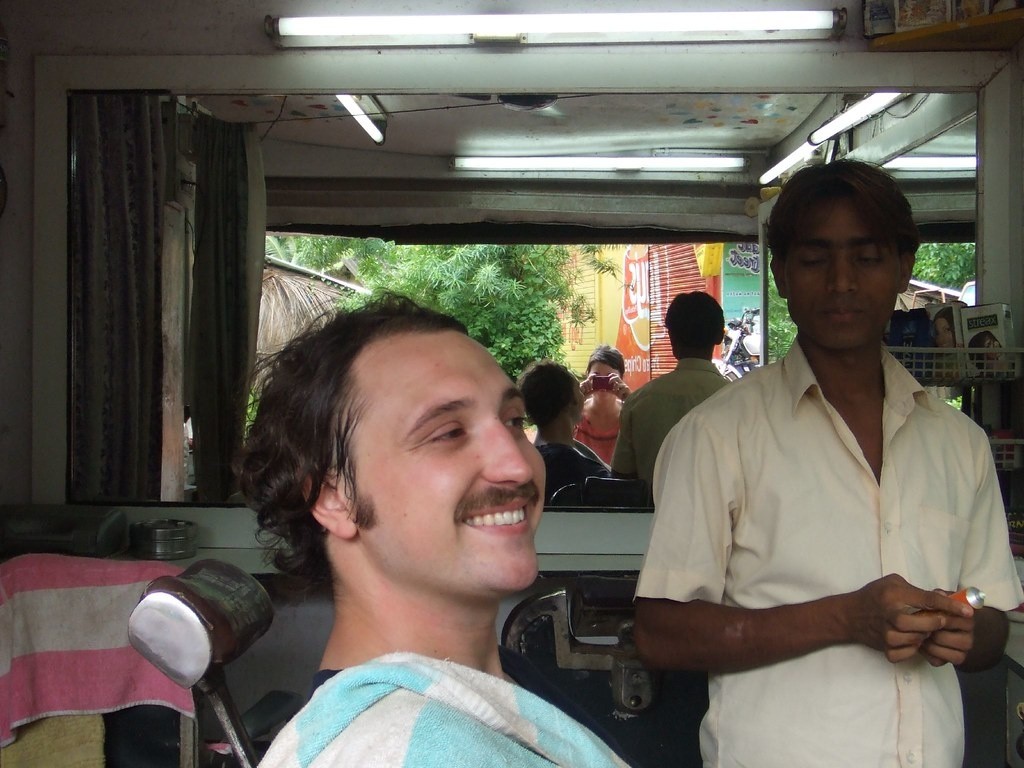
[31,50,1012,555]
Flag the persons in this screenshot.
[573,345,630,466]
[230,293,634,768]
[968,329,1005,378]
[632,158,1024,768]
[932,306,959,378]
[611,291,733,507]
[515,360,613,506]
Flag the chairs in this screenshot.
[0,553,708,768]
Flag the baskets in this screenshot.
[989,439,1024,471]
[882,345,1024,383]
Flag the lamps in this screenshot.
[449,156,752,173]
[757,119,840,187]
[807,92,914,146]
[335,94,389,146]
[263,6,847,50]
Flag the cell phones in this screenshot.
[592,376,613,389]
